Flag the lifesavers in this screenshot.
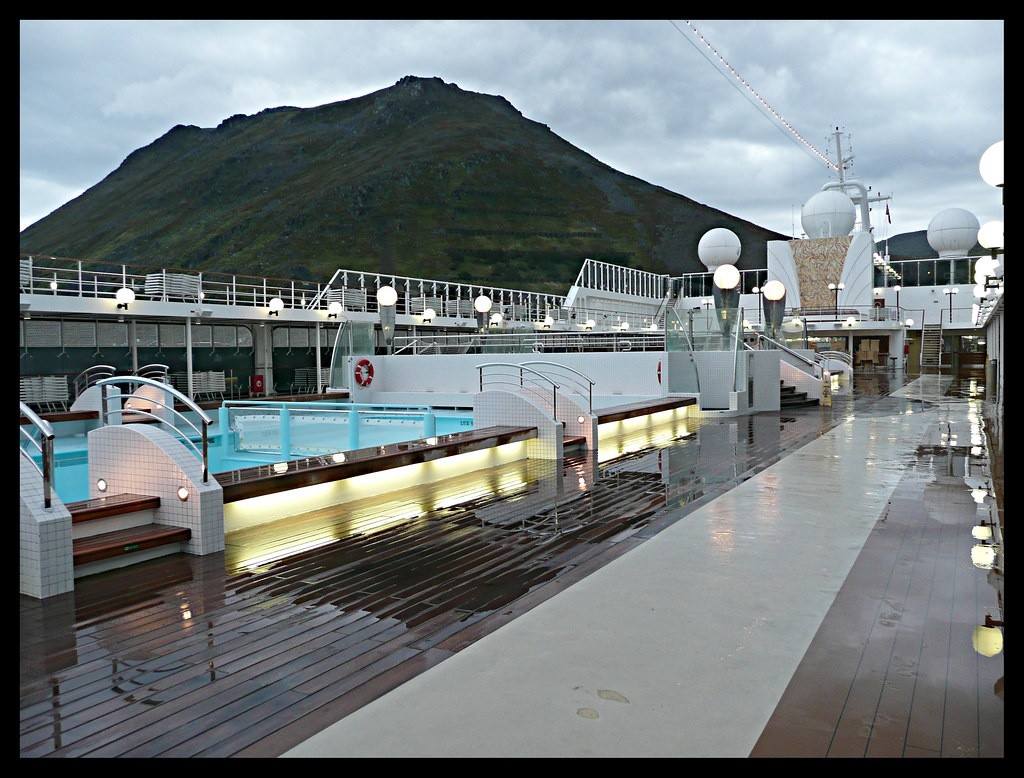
[356,360,375,386]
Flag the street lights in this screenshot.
[943,287,959,324]
[894,285,901,322]
[752,286,766,324]
[828,283,845,321]
[701,298,713,331]
